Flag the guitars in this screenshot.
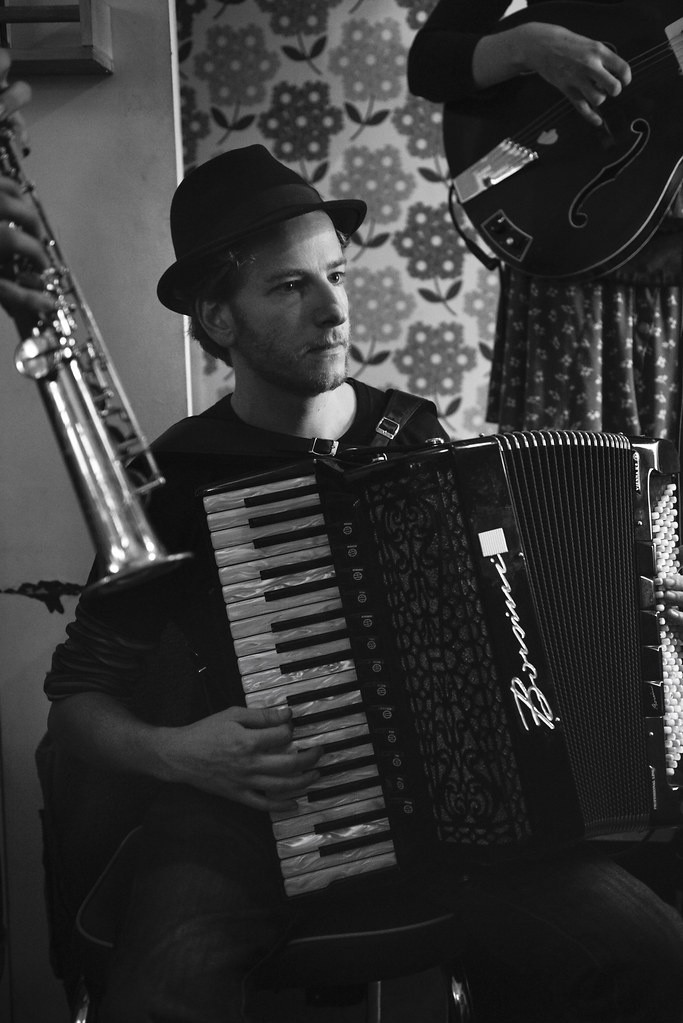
[446,0,681,283]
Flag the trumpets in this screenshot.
[2,105,199,625]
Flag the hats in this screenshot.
[157,144,368,316]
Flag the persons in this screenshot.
[36,144,683,1023]
[408,0,683,433]
[0,45,61,312]
